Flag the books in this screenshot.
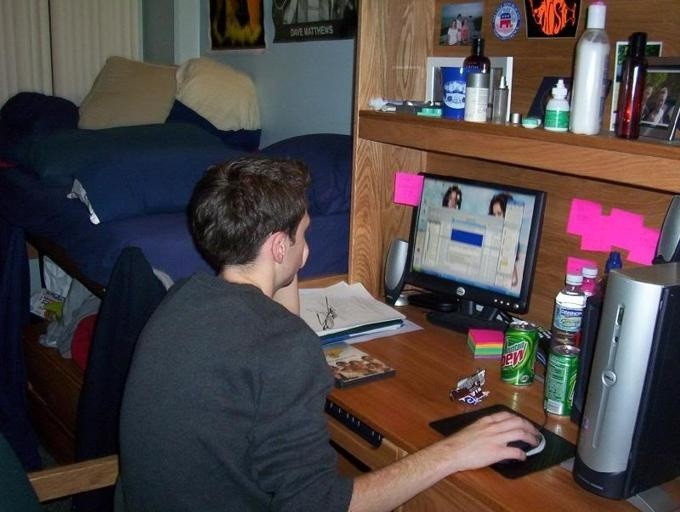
[317,341,396,388]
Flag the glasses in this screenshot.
[317,297,336,330]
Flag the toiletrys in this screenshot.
[617,32,647,139]
[492,89,508,126]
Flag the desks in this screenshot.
[272,277,679,512]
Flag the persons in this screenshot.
[646,86,670,128]
[640,83,654,120]
[490,195,521,285]
[112,156,541,512]
[448,14,476,45]
[330,356,391,381]
[443,185,462,210]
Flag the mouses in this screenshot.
[508,428,545,456]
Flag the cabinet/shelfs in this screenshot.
[349,0,680,332]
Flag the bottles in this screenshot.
[578,263,602,298]
[612,30,649,139]
[551,272,589,355]
[543,77,571,132]
[491,72,509,125]
[603,251,623,285]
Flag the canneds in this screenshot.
[544,343,580,415]
[501,319,540,386]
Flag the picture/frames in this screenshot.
[637,55,680,142]
[423,55,514,121]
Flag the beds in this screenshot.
[0,94,350,311]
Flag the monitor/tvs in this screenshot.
[404,172,549,336]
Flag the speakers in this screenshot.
[384,239,408,308]
[653,194,680,262]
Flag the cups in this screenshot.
[436,65,482,122]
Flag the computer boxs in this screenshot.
[574,263,679,502]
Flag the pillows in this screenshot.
[0,126,75,187]
[77,56,180,130]
[179,57,262,131]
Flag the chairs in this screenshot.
[0,429,120,512]
